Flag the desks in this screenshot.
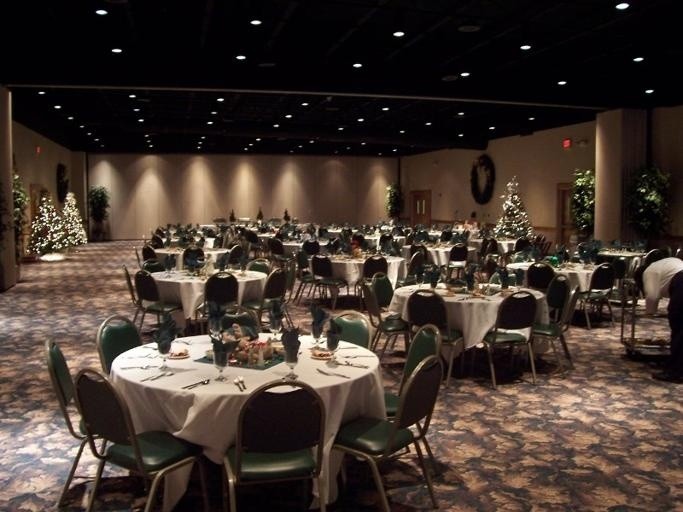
[110,333,379,465]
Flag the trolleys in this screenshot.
[621,278,672,361]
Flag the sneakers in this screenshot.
[654,372,681,383]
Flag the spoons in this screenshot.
[317,369,351,380]
[120,364,150,370]
[234,375,248,393]
[182,379,209,390]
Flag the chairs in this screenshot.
[331,355,444,509]
[222,379,326,510]
[47,338,108,511]
[123,216,682,388]
[73,367,210,510]
[383,325,441,473]
[96,314,144,465]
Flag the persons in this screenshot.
[635,257,683,382]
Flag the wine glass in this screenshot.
[157,341,171,371]
[311,325,323,345]
[417,276,424,289]
[212,350,228,383]
[431,281,437,294]
[326,331,340,368]
[283,349,301,382]
[269,319,282,342]
